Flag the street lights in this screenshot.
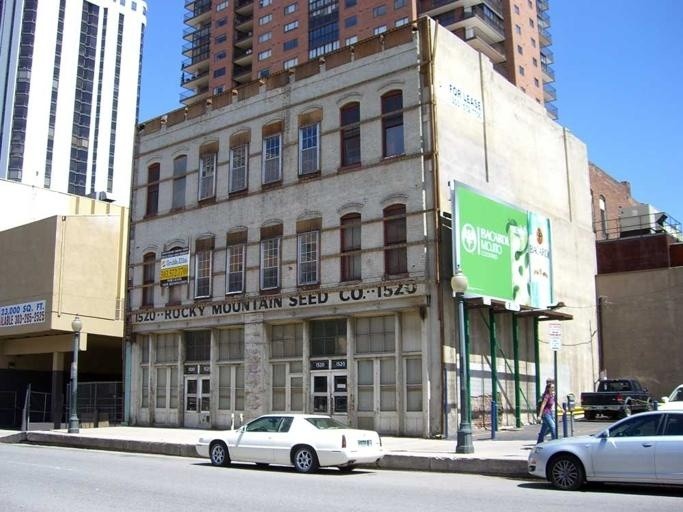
[450,264,474,454]
[68,314,83,433]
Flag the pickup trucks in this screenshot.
[581,377,654,420]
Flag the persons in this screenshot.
[533,383,556,443]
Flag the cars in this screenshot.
[527,383,683,491]
[195,414,384,473]
